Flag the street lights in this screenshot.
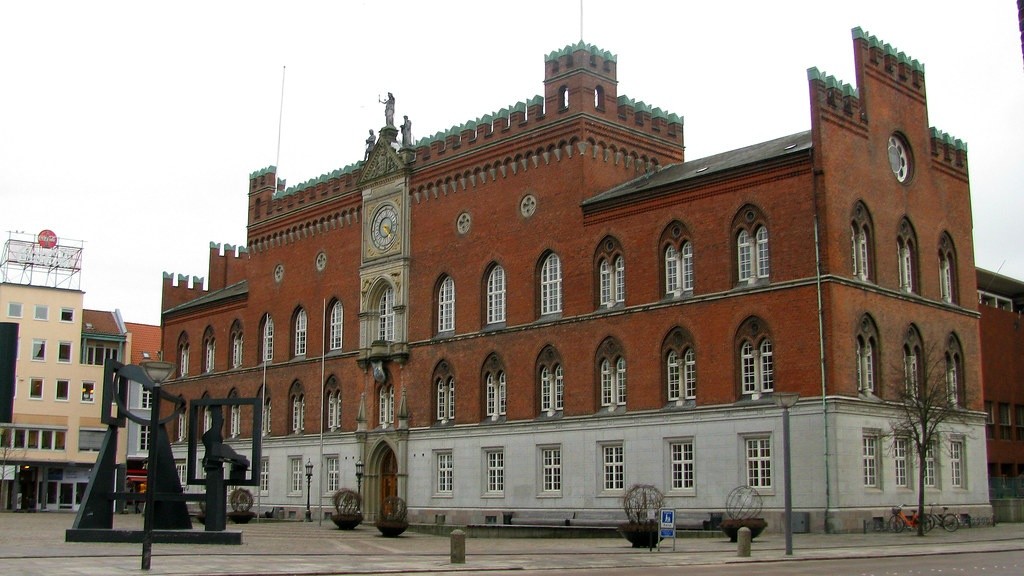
[304,457,314,522]
[354,456,365,513]
[138,359,179,571]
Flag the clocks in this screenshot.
[371,205,399,249]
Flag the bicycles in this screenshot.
[887,504,932,535]
[919,503,960,532]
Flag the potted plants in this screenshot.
[194,501,206,524]
[225,488,256,523]
[374,495,413,536]
[715,485,771,543]
[618,483,666,548]
[329,488,364,529]
